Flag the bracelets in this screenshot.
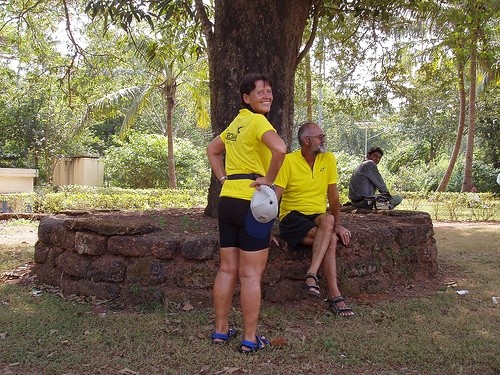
[218,176,228,181]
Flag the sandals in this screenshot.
[328,296,355,319]
[211,328,239,345]
[238,335,271,354]
[304,274,321,296]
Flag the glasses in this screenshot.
[311,134,326,140]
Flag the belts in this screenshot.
[351,199,363,202]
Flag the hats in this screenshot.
[244,184,279,240]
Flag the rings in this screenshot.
[346,233,348,235]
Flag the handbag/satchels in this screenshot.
[225,173,276,191]
[372,196,393,210]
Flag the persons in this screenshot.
[274,123,354,317]
[207,73,287,354]
[349,147,402,210]
